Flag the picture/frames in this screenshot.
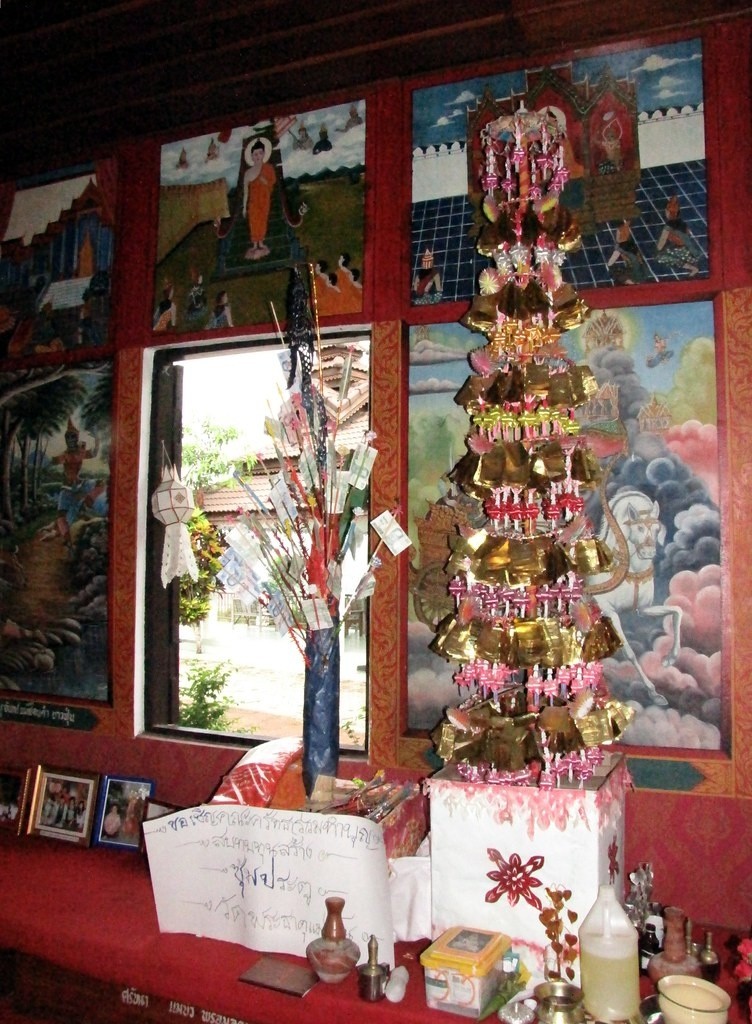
[0,764,185,854]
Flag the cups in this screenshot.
[656,974,730,1024]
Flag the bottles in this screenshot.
[533,982,586,1024]
[639,923,660,975]
[361,934,384,1001]
[647,906,702,983]
[306,896,360,983]
[579,884,641,1021]
[385,965,409,1003]
[700,932,719,983]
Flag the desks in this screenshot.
[0,773,752,1024]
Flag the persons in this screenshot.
[104,805,121,837]
[43,795,85,830]
